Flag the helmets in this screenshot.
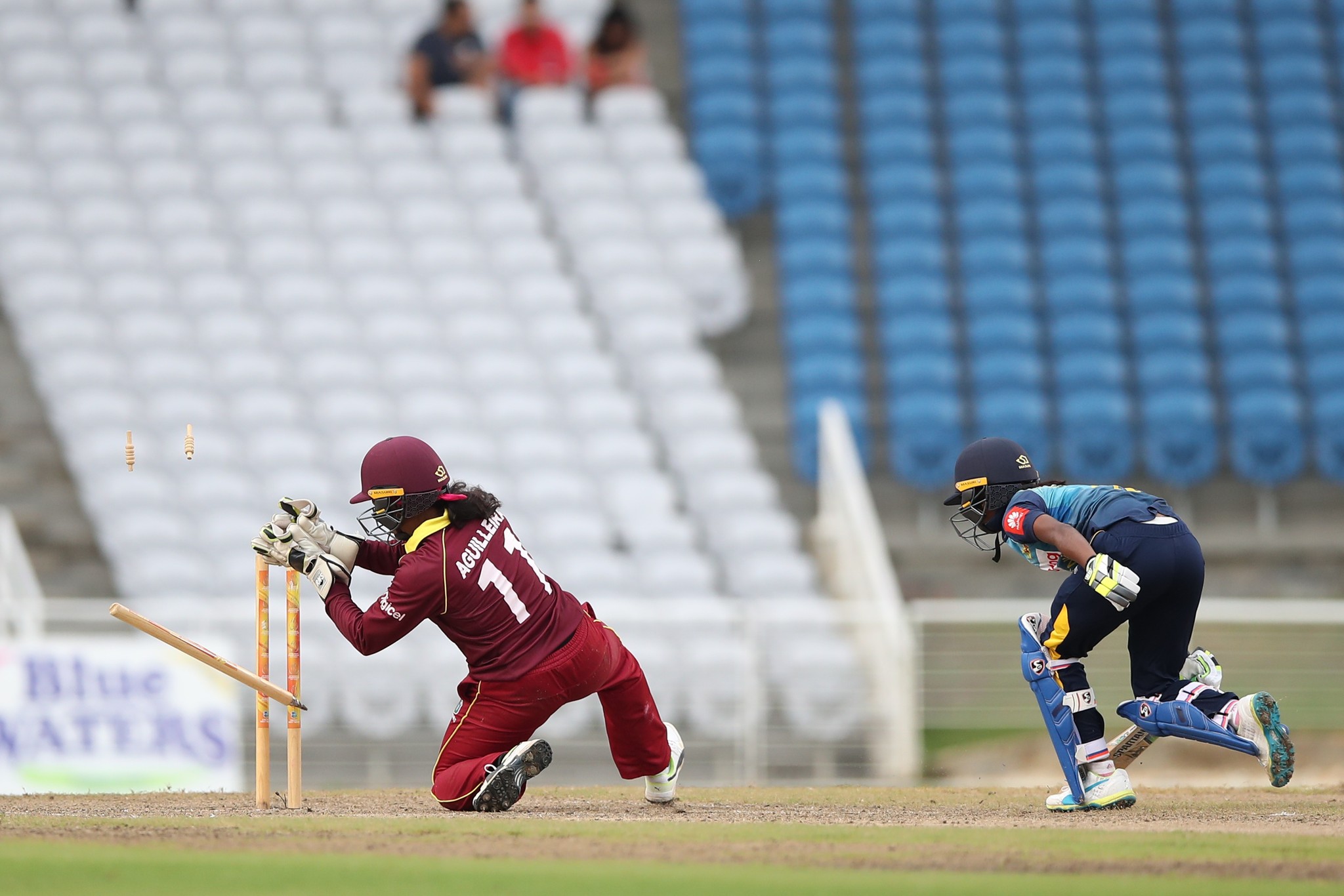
[944,437,1037,506]
[350,436,450,504]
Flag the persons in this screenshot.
[402,1,652,126]
[943,437,1294,812]
[252,435,686,814]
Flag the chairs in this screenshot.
[2,0,1344,789]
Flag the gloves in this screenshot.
[1182,646,1222,693]
[1083,552,1141,612]
[251,522,352,604]
[272,497,366,575]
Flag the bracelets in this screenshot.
[1084,555,1098,574]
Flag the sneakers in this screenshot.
[1045,768,1136,812]
[472,739,554,812]
[1227,691,1295,788]
[644,721,685,804]
